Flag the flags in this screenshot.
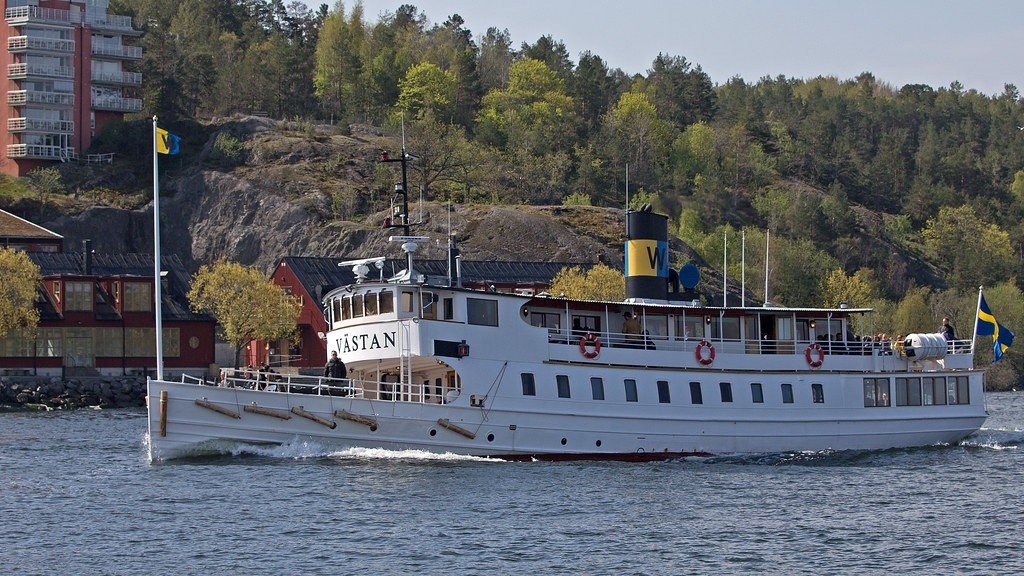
[975,291,1014,365]
[156,127,181,155]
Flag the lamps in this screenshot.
[706,317,711,324]
[810,320,816,328]
[523,308,528,317]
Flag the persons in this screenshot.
[941,318,954,340]
[622,311,657,350]
[218,365,286,392]
[324,351,346,396]
[817,331,905,355]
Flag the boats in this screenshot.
[145,110,991,464]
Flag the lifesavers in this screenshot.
[805,344,824,367]
[579,334,600,358]
[696,340,715,365]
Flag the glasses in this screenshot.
[332,354,337,356]
